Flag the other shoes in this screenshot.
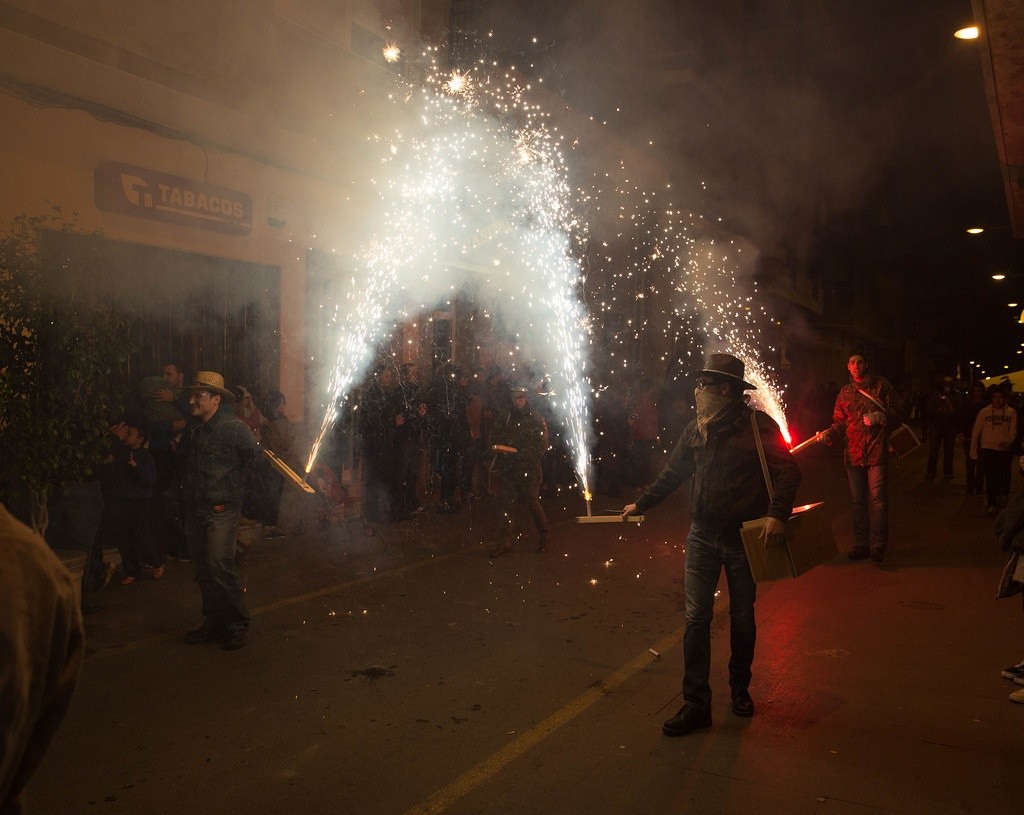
[870,548,882,561]
[223,622,249,649]
[489,542,513,557]
[987,504,998,513]
[263,525,286,540]
[999,494,1009,505]
[184,618,216,643]
[393,497,458,521]
[1001,661,1024,703]
[538,530,553,553]
[848,545,870,560]
[120,576,136,584]
[94,561,116,592]
[153,564,168,579]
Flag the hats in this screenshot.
[942,376,954,387]
[840,336,876,368]
[509,379,529,397]
[692,353,757,389]
[180,371,235,400]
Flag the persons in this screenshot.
[891,375,1024,703]
[89,354,292,588]
[0,502,88,813]
[813,380,839,423]
[583,365,689,499]
[616,354,803,735]
[174,369,262,650]
[813,348,910,565]
[356,360,571,519]
[484,379,554,556]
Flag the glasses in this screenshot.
[696,381,720,389]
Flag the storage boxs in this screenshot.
[739,501,841,583]
[887,423,922,459]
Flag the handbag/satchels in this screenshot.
[887,425,922,459]
[738,503,839,582]
[480,460,500,493]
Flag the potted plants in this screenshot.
[0,202,172,635]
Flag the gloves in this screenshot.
[863,413,881,425]
[816,431,832,444]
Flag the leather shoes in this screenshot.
[663,706,712,735]
[731,687,753,717]
